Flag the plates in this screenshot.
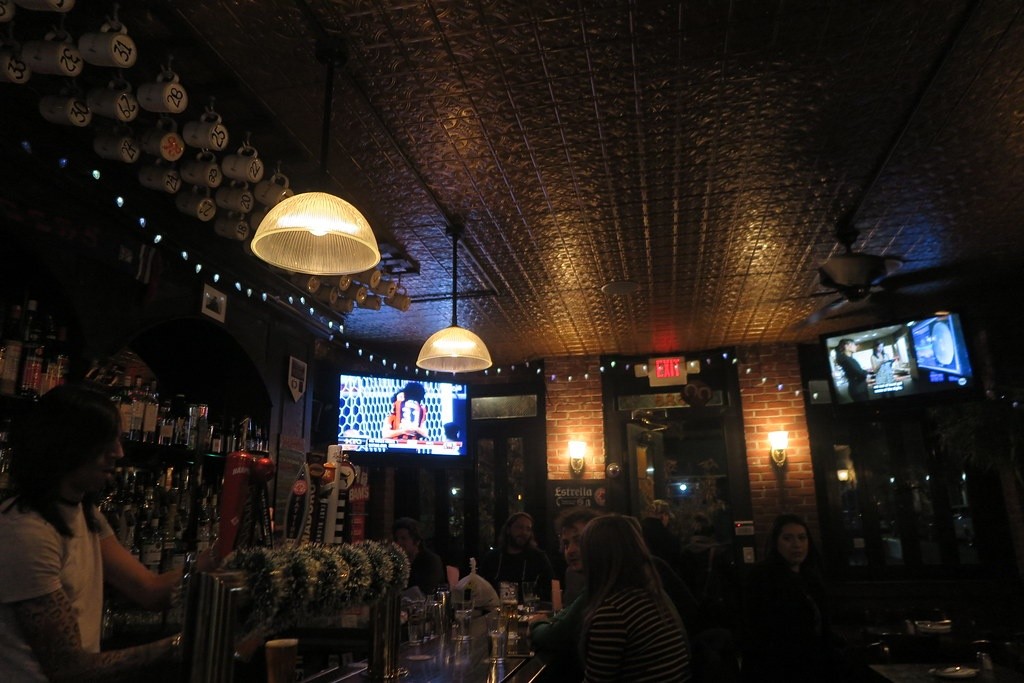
[935,667,976,678]
[915,621,951,633]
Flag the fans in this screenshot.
[746,227,973,331]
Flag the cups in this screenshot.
[407,582,537,664]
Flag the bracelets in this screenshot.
[206,538,220,568]
[173,632,185,661]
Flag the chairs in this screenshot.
[689,628,740,683]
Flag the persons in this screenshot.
[0,384,224,683]
[831,337,899,398]
[381,382,429,442]
[386,505,831,683]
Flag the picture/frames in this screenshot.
[198,280,231,328]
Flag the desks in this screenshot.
[867,662,1024,683]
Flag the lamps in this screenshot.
[415,223,492,373]
[252,38,380,274]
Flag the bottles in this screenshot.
[0,300,269,572]
